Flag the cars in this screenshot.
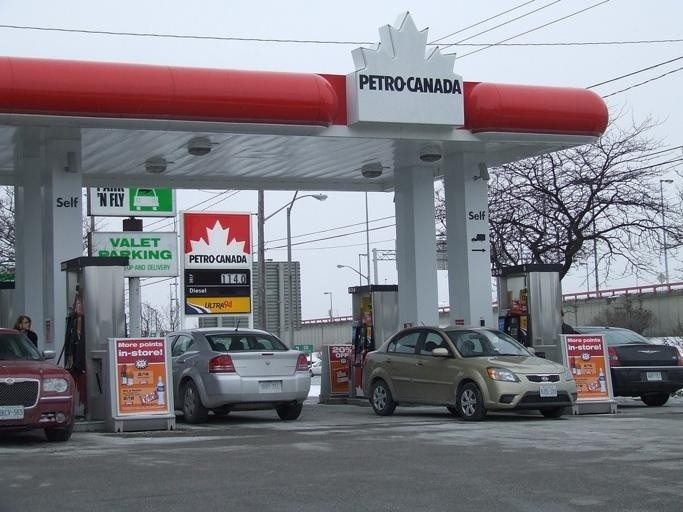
[569,322,681,409]
[308,360,322,378]
[162,326,310,423]
[362,326,575,419]
[0,327,73,443]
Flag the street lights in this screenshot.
[337,262,370,288]
[323,291,334,322]
[359,252,368,288]
[579,261,591,299]
[259,192,327,337]
[659,176,674,286]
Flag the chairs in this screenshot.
[459,341,481,356]
[424,341,439,352]
[214,341,266,351]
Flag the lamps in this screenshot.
[361,166,383,178]
[188,142,211,158]
[420,149,442,163]
[145,162,168,173]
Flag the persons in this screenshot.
[13,314,38,351]
[561,309,575,334]
